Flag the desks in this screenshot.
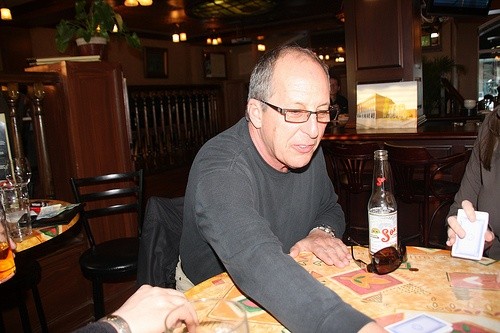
[177,246,499,332]
[12,198,82,259]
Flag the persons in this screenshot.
[330,77,348,117]
[445,107,500,261]
[175,47,388,333]
[0,224,198,333]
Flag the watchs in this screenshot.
[311,223,335,237]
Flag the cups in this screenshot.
[0,182,32,243]
[164,297,249,333]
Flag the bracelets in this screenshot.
[101,314,131,333]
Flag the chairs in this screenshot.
[138,196,191,291]
[69,169,146,323]
[0,258,51,333]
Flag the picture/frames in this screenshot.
[142,45,169,80]
[203,52,228,81]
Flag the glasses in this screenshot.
[258,99,339,122]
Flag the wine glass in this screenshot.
[4,157,32,208]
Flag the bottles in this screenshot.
[0,201,17,283]
[367,149,398,260]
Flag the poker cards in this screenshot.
[385,314,451,333]
[452,209,490,261]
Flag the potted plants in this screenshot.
[54,0,143,57]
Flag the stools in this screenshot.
[323,140,471,248]
